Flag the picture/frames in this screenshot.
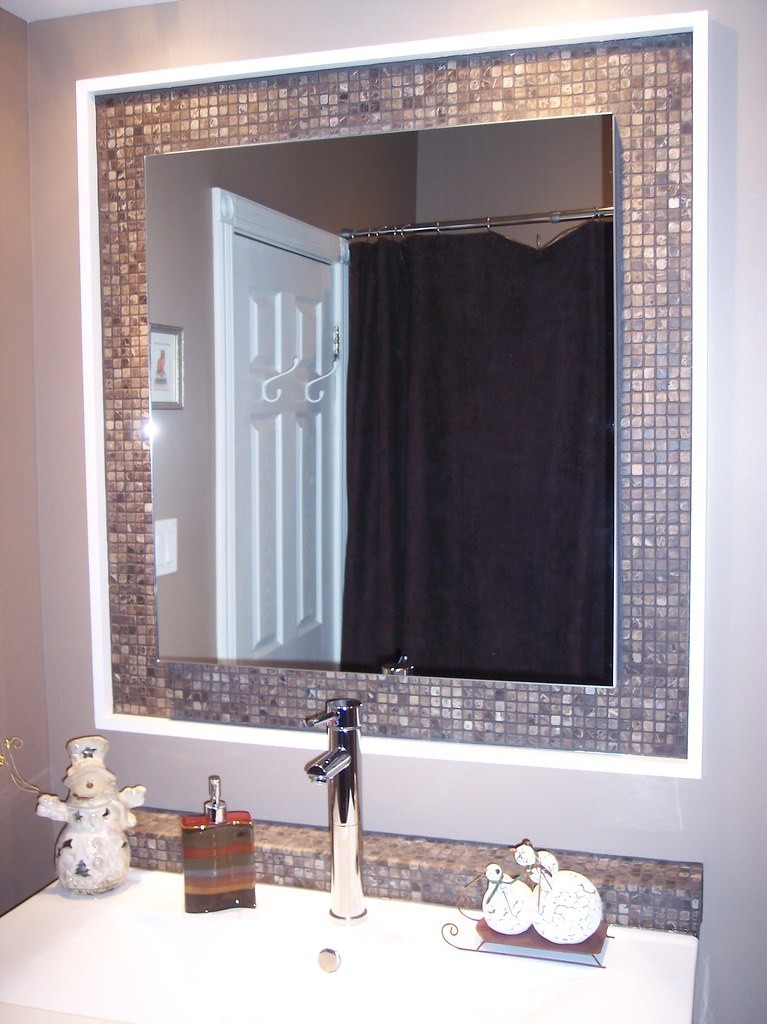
[149,322,185,411]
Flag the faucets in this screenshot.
[383,652,413,674]
[304,697,368,918]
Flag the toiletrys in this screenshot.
[180,774,257,914]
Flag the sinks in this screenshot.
[1,905,699,1024]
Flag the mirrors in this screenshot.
[142,112,619,692]
